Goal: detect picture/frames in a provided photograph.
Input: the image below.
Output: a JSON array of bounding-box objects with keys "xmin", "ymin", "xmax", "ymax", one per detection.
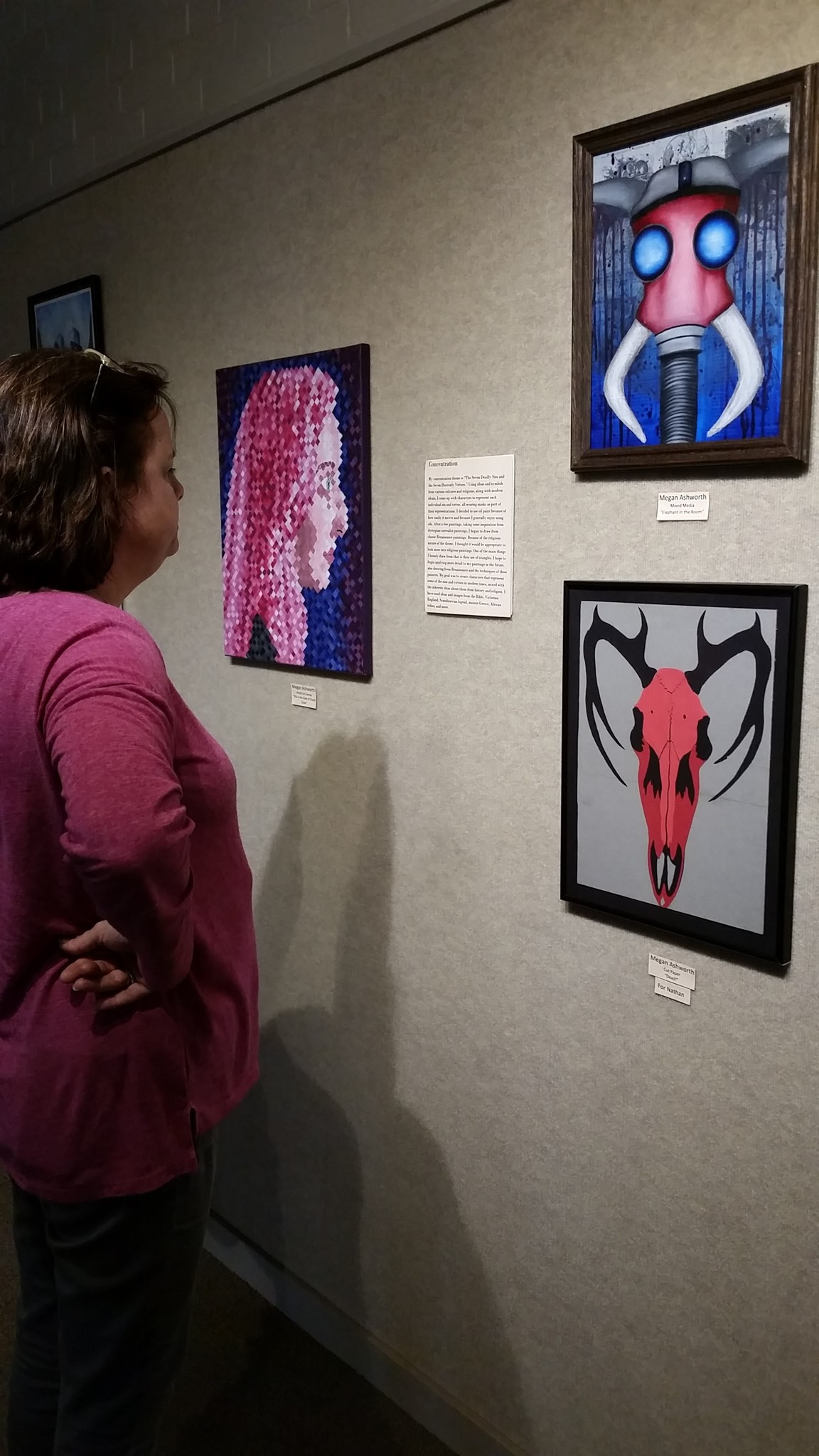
[
  {"xmin": 28, "ymin": 274, "xmax": 106, "ymax": 356},
  {"xmin": 558, "ymin": 578, "xmax": 809, "ymax": 967},
  {"xmin": 572, "ymin": 62, "xmax": 819, "ymax": 473}
]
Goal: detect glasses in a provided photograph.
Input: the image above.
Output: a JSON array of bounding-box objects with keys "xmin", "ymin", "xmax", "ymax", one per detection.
[{"xmin": 82, "ymin": 346, "xmax": 130, "ymax": 398}]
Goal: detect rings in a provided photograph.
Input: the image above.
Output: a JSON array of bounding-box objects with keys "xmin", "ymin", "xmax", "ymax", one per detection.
[{"xmin": 126, "ymin": 969, "xmax": 134, "ymax": 984}]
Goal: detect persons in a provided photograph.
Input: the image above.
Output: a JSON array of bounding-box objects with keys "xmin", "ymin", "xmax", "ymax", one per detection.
[{"xmin": 0, "ymin": 341, "xmax": 262, "ymax": 1456}]
